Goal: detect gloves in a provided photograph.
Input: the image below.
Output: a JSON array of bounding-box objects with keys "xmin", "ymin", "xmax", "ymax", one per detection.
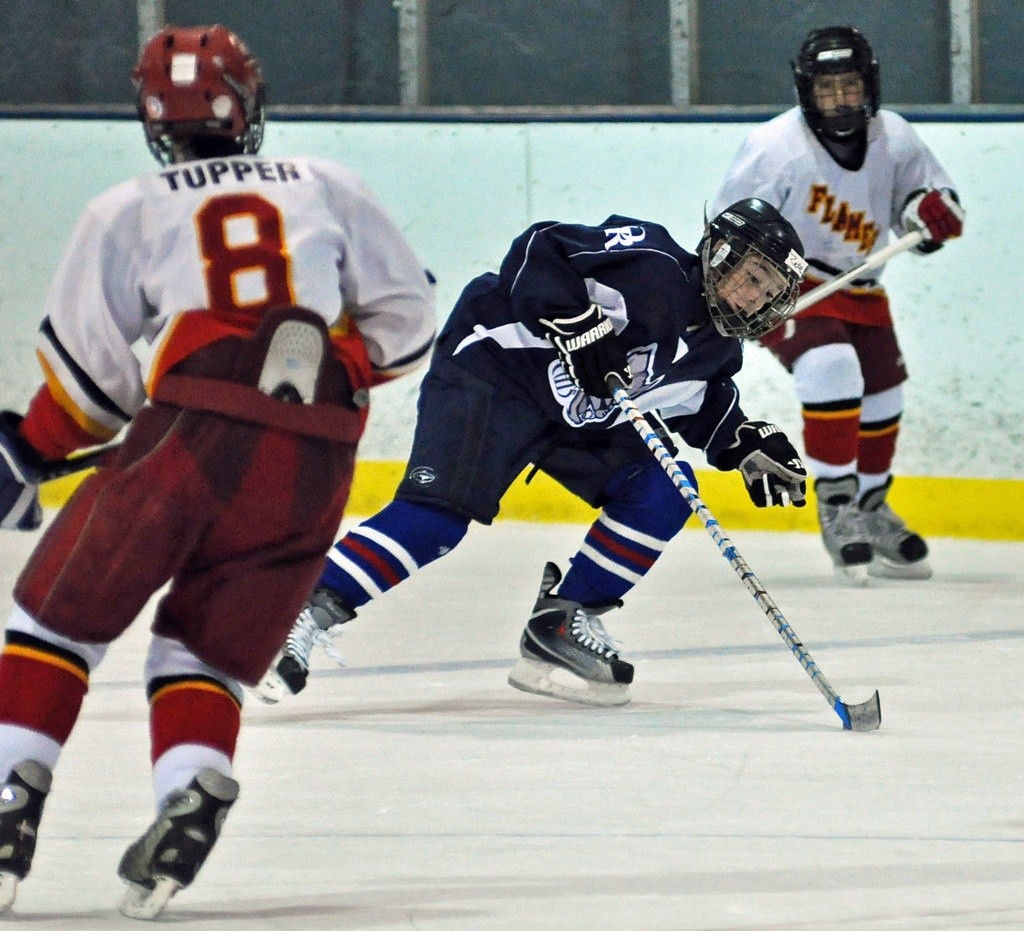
[
  {"xmin": 538, "ymin": 301, "xmax": 634, "ymax": 399},
  {"xmin": 915, "ymin": 188, "xmax": 964, "ymax": 241},
  {"xmin": 0, "ymin": 410, "xmax": 42, "ymax": 531},
  {"xmin": 724, "ymin": 421, "xmax": 807, "ymax": 508}
]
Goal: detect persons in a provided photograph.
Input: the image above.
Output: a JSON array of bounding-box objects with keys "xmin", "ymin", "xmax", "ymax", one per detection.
[
  {"xmin": 0, "ymin": 24, "xmax": 438, "ymax": 918},
  {"xmin": 706, "ymin": 24, "xmax": 965, "ymax": 586},
  {"xmin": 240, "ymin": 197, "xmax": 806, "ymax": 708}
]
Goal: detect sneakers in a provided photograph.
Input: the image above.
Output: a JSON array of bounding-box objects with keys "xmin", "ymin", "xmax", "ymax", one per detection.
[
  {"xmin": 508, "ymin": 562, "xmax": 633, "ymax": 706},
  {"xmin": 116, "ymin": 768, "xmax": 239, "ymax": 917},
  {"xmin": 815, "ymin": 474, "xmax": 875, "ymax": 587},
  {"xmin": 0, "ymin": 759, "xmax": 52, "ymax": 912},
  {"xmin": 240, "ymin": 579, "xmax": 357, "ymax": 705},
  {"xmin": 856, "ymin": 474, "xmax": 934, "ymax": 580}
]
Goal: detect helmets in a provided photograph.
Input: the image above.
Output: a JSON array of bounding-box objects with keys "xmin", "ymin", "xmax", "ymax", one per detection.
[
  {"xmin": 791, "ymin": 26, "xmax": 879, "ymax": 131},
  {"xmin": 695, "ymin": 197, "xmax": 808, "ymax": 335},
  {"xmin": 132, "ymin": 24, "xmax": 265, "ymax": 165}
]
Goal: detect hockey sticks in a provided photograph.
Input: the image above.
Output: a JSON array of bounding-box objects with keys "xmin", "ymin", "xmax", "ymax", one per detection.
[
  {"xmin": 786, "ymin": 229, "xmax": 921, "ymax": 317},
  {"xmin": 34, "ymin": 443, "xmax": 122, "ymax": 486},
  {"xmin": 607, "ymin": 369, "xmax": 884, "ymax": 733}
]
